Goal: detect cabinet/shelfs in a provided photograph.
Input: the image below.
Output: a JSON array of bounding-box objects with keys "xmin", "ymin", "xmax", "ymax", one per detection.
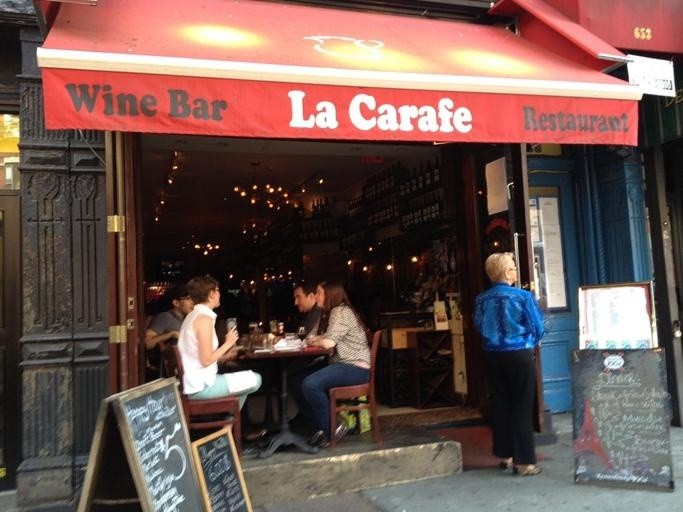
[
  {"xmin": 379, "ymin": 312, "xmax": 433, "ymax": 407},
  {"xmin": 407, "ymin": 330, "xmax": 457, "ymax": 409}
]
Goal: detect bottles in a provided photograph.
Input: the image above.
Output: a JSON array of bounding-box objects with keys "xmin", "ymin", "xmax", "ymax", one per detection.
[
  {"xmin": 297, "ymin": 156, "xmax": 443, "ymax": 249},
  {"xmin": 278, "ymin": 321, "xmax": 285, "ymax": 337}
]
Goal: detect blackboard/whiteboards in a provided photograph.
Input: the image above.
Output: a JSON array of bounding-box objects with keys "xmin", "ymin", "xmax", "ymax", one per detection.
[
  {"xmin": 570, "ymin": 347, "xmax": 674, "ymax": 492},
  {"xmin": 112, "ymin": 376, "xmax": 207, "ymax": 511},
  {"xmin": 191, "ymin": 424, "xmax": 254, "ymax": 512}
]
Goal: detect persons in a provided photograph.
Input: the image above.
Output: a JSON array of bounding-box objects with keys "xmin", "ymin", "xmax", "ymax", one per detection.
[
  {"xmin": 144, "ymin": 286, "xmax": 269, "ymax": 441},
  {"xmin": 299, "ymin": 281, "xmax": 371, "ymax": 449},
  {"xmin": 284, "ymin": 279, "xmax": 324, "ymax": 420},
  {"xmin": 473, "ymin": 251, "xmax": 546, "ymax": 475},
  {"xmin": 178, "ymin": 277, "xmax": 263, "ymax": 430}
]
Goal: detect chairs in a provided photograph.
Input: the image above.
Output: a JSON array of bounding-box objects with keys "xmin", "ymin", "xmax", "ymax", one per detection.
[
  {"xmin": 172, "ymin": 344, "xmax": 242, "ymax": 460},
  {"xmin": 329, "ymin": 330, "xmax": 382, "ymax": 447},
  {"xmin": 145, "ymin": 342, "xmax": 164, "ymax": 375}
]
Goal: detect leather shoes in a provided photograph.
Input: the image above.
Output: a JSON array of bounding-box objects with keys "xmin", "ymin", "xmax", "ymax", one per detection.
[
  {"xmin": 310, "ymin": 429, "xmax": 324, "ymax": 446},
  {"xmin": 513, "ymin": 464, "xmax": 541, "ymax": 476},
  {"xmin": 321, "ymin": 424, "xmax": 347, "ymax": 447},
  {"xmin": 501, "ymin": 458, "xmax": 513, "ymax": 471}
]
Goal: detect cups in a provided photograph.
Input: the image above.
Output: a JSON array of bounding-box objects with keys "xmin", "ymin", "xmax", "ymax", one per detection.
[
  {"xmin": 225, "ymin": 318, "xmax": 240, "ymax": 347},
  {"xmin": 424, "ymin": 319, "xmax": 434, "ymax": 331},
  {"xmin": 286, "ymin": 333, "xmax": 296, "ymax": 347},
  {"xmin": 248, "ymin": 321, "xmax": 259, "ymax": 342},
  {"xmin": 269, "ymin": 320, "xmax": 278, "ymax": 336},
  {"xmin": 262, "ymin": 336, "xmax": 272, "ymax": 351}
]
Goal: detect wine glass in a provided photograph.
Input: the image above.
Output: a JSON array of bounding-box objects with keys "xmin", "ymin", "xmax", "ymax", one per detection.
[{"xmin": 298, "ymin": 327, "xmax": 308, "ymax": 345}]
